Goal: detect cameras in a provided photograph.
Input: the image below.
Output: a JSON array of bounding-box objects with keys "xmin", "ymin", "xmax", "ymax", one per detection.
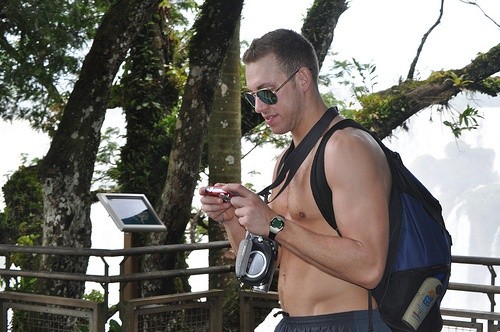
[{"xmin": 236, "ymin": 235, "xmax": 280, "ymax": 294}]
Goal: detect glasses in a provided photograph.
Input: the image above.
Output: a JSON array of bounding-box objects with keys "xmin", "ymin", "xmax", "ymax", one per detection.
[{"xmin": 244, "ymin": 69, "xmax": 301, "ymax": 108}]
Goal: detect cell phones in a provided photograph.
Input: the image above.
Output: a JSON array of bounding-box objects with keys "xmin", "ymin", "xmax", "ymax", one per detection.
[{"xmin": 205, "ymin": 187, "xmax": 241, "ymax": 202}]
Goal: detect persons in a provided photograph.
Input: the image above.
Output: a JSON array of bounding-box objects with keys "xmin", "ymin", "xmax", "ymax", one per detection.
[{"xmin": 199, "ymin": 29, "xmax": 392, "ymax": 332}]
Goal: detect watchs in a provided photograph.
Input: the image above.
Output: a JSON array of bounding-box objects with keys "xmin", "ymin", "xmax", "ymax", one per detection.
[{"xmin": 268, "ymin": 214, "xmax": 285, "ymax": 240}]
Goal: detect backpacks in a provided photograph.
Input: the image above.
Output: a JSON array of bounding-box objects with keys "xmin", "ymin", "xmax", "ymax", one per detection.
[{"xmin": 310, "ymin": 118, "xmax": 453, "ymax": 332}]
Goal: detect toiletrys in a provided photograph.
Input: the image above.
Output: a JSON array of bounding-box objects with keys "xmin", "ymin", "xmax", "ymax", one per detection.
[{"xmin": 402, "ymin": 274, "xmax": 445, "ymax": 330}]
